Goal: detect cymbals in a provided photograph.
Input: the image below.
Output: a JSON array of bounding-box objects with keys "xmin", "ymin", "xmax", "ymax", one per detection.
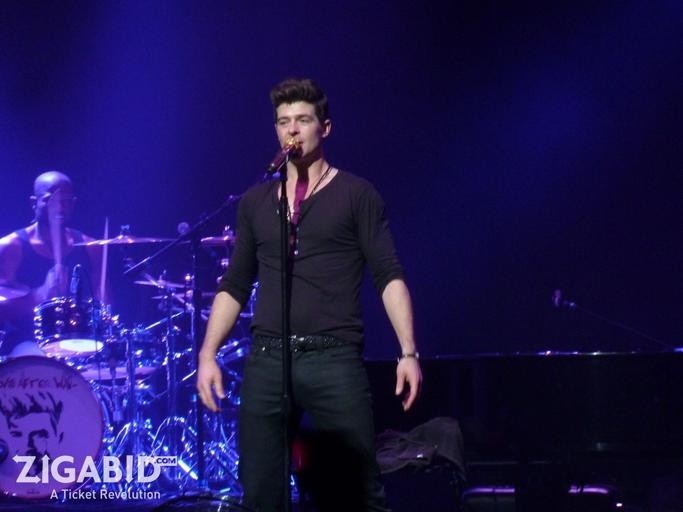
[
  {"xmin": 152, "ymin": 292, "xmax": 215, "ymax": 299},
  {"xmin": 72, "ymin": 236, "xmax": 174, "ymax": 246},
  {"xmin": 182, "ymin": 235, "xmax": 237, "ymax": 245},
  {"xmin": 134, "ymin": 279, "xmax": 186, "ymax": 288}
]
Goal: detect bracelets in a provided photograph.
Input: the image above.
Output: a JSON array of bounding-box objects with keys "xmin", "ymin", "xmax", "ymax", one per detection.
[{"xmin": 396, "ymin": 350, "xmax": 421, "ymax": 360}]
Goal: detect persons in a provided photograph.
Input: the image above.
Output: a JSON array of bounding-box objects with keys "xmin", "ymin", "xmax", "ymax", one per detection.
[
  {"xmin": 0, "ymin": 169, "xmax": 109, "ymax": 357},
  {"xmin": 193, "ymin": 78, "xmax": 424, "ymax": 511}
]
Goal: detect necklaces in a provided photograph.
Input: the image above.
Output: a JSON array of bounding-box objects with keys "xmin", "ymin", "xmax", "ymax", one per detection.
[{"xmin": 269, "ymin": 163, "xmax": 331, "ymax": 228}]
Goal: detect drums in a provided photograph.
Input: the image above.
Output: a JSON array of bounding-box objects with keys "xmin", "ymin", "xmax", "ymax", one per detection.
[
  {"xmin": 35, "ymin": 297, "xmax": 106, "ymax": 357},
  {"xmin": 1, "ymin": 353, "xmax": 105, "ymax": 501},
  {"xmin": 69, "ymin": 324, "xmax": 165, "ymax": 380}
]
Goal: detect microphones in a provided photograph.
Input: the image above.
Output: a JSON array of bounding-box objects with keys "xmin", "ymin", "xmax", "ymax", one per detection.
[
  {"xmin": 552, "ymin": 289, "xmax": 563, "ymax": 308},
  {"xmin": 259, "ymin": 138, "xmax": 301, "ymax": 185},
  {"xmin": 69, "ymin": 264, "xmax": 82, "ymax": 294}
]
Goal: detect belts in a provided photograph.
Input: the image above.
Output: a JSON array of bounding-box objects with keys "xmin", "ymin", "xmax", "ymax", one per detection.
[{"xmin": 257, "ymin": 335, "xmax": 338, "ymax": 352}]
